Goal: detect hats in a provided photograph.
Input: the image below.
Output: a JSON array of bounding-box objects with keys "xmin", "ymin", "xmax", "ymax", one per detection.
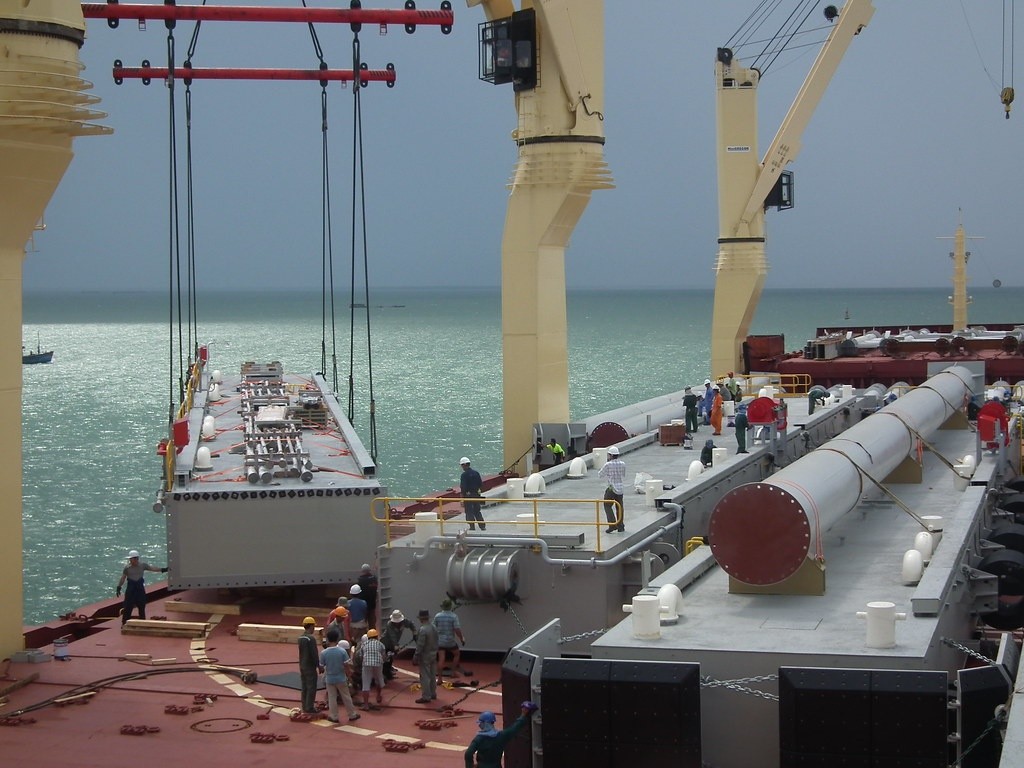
[
  {"xmin": 126, "ymin": 550, "xmax": 138, "ymax": 559},
  {"xmin": 336, "ymin": 597, "xmax": 350, "ymax": 607},
  {"xmin": 417, "ymin": 609, "xmax": 430, "ymax": 618},
  {"xmin": 739, "ymin": 405, "xmax": 746, "ymax": 410},
  {"xmin": 390, "ymin": 610, "xmax": 404, "ymax": 623},
  {"xmin": 706, "ymin": 440, "xmax": 714, "ymax": 447},
  {"xmin": 728, "ymin": 372, "xmax": 734, "ymax": 375}
]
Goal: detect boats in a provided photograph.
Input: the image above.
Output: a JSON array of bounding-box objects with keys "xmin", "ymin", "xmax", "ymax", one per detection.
[{"xmin": 21, "ymin": 346, "xmax": 55, "ymax": 364}]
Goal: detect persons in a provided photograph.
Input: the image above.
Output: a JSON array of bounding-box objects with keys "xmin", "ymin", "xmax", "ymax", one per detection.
[
  {"xmin": 297, "ymin": 617, "xmax": 324, "ymax": 713},
  {"xmin": 598, "ymin": 446, "xmax": 625, "ymax": 533},
  {"xmin": 116, "ymin": 550, "xmax": 172, "ymax": 629},
  {"xmin": 682, "ymin": 386, "xmax": 699, "ymax": 433},
  {"xmin": 320, "ymin": 564, "xmax": 418, "ymax": 711},
  {"xmin": 412, "ymin": 609, "xmax": 439, "ymax": 703},
  {"xmin": 734, "ymin": 405, "xmax": 752, "ymax": 454},
  {"xmin": 710, "ymin": 385, "xmax": 723, "ymax": 436},
  {"xmin": 432, "ymin": 599, "xmax": 465, "ymax": 685},
  {"xmin": 716, "ymin": 372, "xmax": 743, "ymax": 404},
  {"xmin": 464, "ymin": 705, "xmax": 531, "ymax": 768},
  {"xmin": 808, "ymin": 389, "xmax": 830, "ymax": 415},
  {"xmin": 698, "ymin": 380, "xmax": 716, "ymax": 425},
  {"xmin": 538, "ymin": 438, "xmax": 565, "ymax": 466},
  {"xmin": 319, "ymin": 630, "xmax": 361, "ymax": 722},
  {"xmin": 700, "ymin": 440, "xmax": 718, "ymax": 468},
  {"xmin": 459, "ymin": 457, "xmax": 487, "ymax": 531}
]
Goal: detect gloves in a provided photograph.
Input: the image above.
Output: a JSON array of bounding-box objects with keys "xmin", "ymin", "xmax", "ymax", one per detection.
[
  {"xmin": 116, "ymin": 586, "xmax": 121, "ymax": 597},
  {"xmin": 161, "ymin": 567, "xmax": 168, "ymax": 572}
]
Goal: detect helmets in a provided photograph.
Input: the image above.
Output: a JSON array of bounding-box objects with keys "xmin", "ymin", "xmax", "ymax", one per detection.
[
  {"xmin": 337, "ymin": 640, "xmax": 350, "ymax": 649},
  {"xmin": 608, "ymin": 447, "xmax": 620, "ymax": 455},
  {"xmin": 460, "ymin": 457, "xmax": 470, "ymax": 464},
  {"xmin": 367, "ymin": 629, "xmax": 379, "ymax": 638},
  {"xmin": 335, "ymin": 606, "xmax": 348, "ymax": 618},
  {"xmin": 303, "ymin": 617, "xmax": 318, "ymax": 626},
  {"xmin": 713, "ymin": 386, "xmax": 719, "ymax": 390},
  {"xmin": 705, "ymin": 380, "xmax": 710, "ymax": 384},
  {"xmin": 361, "ymin": 634, "xmax": 368, "ymax": 642},
  {"xmin": 350, "ymin": 585, "xmax": 363, "ymax": 594}
]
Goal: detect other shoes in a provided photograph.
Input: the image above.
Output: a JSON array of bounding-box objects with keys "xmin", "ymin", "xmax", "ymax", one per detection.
[
  {"xmin": 308, "ymin": 667, "xmax": 438, "ymax": 722},
  {"xmin": 606, "ymin": 526, "xmax": 618, "ymax": 533},
  {"xmin": 618, "ymin": 527, "xmax": 625, "ymax": 532}
]
